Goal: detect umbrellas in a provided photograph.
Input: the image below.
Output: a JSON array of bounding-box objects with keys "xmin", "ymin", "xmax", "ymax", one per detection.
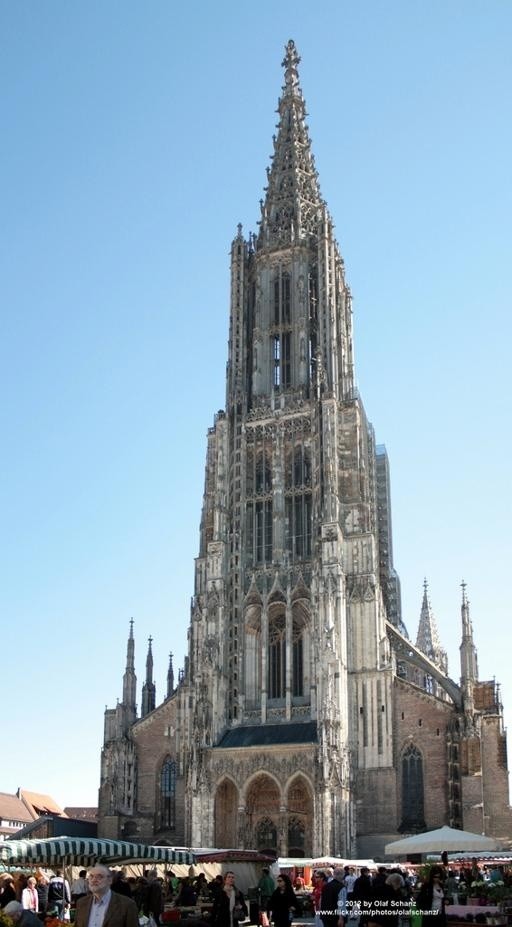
[{"xmin": 384, "ymin": 825, "xmax": 498, "ymax": 864}]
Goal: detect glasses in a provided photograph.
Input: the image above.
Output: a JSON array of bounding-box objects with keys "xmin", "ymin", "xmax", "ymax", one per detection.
[
  {"xmin": 276, "ymin": 879, "xmax": 286, "ymax": 884},
  {"xmin": 88, "ymin": 874, "xmax": 109, "ymax": 881}
]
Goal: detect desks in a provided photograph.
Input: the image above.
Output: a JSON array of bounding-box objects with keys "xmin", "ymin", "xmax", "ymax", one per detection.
[{"xmin": 445, "ymin": 905, "xmax": 500, "ymax": 918}]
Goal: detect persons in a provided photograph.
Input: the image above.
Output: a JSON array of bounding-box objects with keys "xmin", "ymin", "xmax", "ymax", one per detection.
[{"xmin": 1, "ymin": 862, "xmax": 512, "ymax": 927}]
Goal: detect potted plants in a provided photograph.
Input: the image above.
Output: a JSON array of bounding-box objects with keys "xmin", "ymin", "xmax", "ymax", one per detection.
[{"xmin": 443, "ymin": 878, "xmax": 512, "ymax": 910}]
